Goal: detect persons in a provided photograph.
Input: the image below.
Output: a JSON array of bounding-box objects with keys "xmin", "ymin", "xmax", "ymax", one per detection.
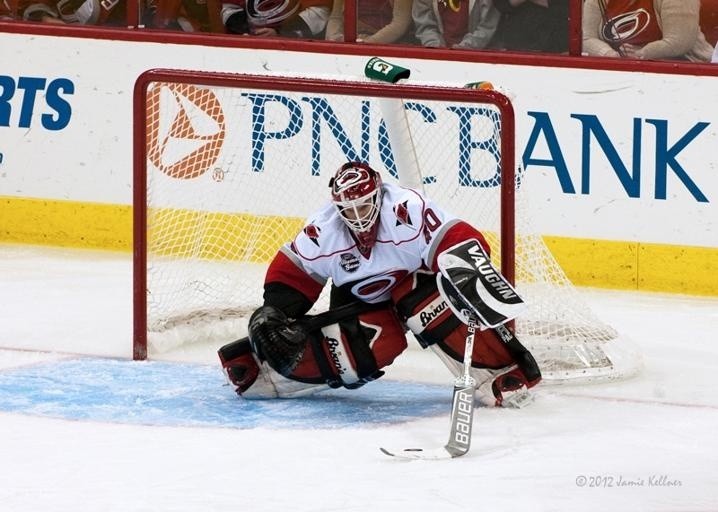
[
  {"xmin": 216, "ymin": 162, "xmax": 543, "ymax": 412},
  {"xmin": 0, "ymin": 0, "xmax": 718, "ymax": 68}
]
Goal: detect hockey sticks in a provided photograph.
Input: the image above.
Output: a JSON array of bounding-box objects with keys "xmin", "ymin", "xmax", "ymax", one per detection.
[{"xmin": 378, "ymin": 313, "xmax": 477, "ymax": 461}]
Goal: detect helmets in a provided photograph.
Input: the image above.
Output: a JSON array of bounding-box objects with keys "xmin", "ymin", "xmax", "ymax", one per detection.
[{"xmin": 331, "ymin": 163, "xmax": 381, "ymax": 248}]
